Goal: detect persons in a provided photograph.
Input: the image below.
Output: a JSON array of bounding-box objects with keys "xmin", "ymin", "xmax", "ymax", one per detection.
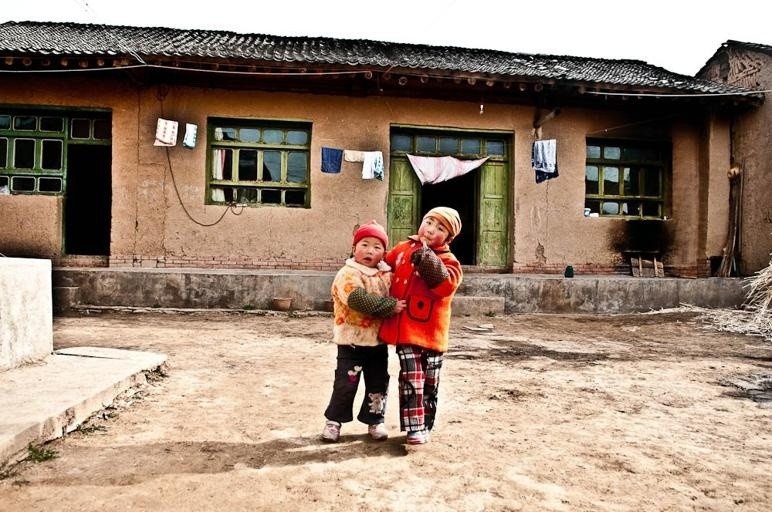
[
  {"xmin": 384, "ymin": 206, "xmax": 463, "ymax": 444},
  {"xmin": 322, "ymin": 221, "xmax": 407, "ymax": 441}
]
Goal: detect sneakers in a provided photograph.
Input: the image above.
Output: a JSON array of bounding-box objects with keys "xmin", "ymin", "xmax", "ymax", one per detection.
[
  {"xmin": 406, "ymin": 430, "xmax": 431, "ymax": 444},
  {"xmin": 367, "ymin": 423, "xmax": 388, "ymax": 440},
  {"xmin": 322, "ymin": 421, "xmax": 341, "ymax": 442}
]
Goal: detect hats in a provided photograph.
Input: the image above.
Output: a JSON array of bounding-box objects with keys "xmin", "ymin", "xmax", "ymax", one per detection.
[
  {"xmin": 423, "ymin": 207, "xmax": 462, "ymax": 236},
  {"xmin": 353, "ymin": 220, "xmax": 388, "ymax": 250}
]
